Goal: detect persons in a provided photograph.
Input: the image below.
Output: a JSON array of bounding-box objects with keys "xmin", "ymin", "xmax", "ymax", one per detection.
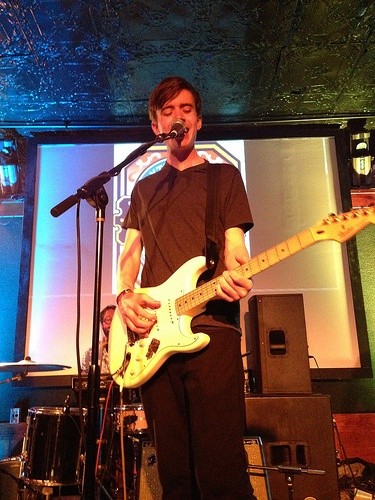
[
  {"xmin": 116, "ymin": 77, "xmax": 258, "ymax": 500},
  {"xmin": 62, "ymin": 305, "xmax": 118, "ymax": 478}
]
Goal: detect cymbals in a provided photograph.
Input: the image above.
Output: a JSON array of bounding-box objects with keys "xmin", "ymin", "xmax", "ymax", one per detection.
[{"xmin": 0, "ymin": 356, "xmax": 72, "ymax": 373}]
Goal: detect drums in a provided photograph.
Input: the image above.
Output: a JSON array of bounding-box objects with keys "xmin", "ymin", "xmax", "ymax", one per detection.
[
  {"xmin": 111, "ymin": 386, "xmax": 150, "ymax": 433},
  {"xmin": 19, "ymin": 407, "xmax": 88, "ymax": 486}
]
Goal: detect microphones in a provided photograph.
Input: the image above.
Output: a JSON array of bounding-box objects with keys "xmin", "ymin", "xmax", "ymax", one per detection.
[{"xmin": 169, "ymin": 119, "xmax": 184, "ymax": 138}]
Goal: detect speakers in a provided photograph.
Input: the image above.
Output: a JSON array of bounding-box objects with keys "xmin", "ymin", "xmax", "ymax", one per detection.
[
  {"xmin": 115, "ymin": 429, "xmax": 270, "ymax": 500},
  {"xmin": 248, "ymin": 292, "xmax": 313, "ymax": 396},
  {"xmin": 242, "ymin": 393, "xmax": 340, "ymax": 500}
]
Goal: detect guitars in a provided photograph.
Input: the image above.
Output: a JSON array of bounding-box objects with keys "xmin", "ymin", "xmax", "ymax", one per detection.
[{"xmin": 108, "ymin": 208, "xmax": 375, "ymax": 385}]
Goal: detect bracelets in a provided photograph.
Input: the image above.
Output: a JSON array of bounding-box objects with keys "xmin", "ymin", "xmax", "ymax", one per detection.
[{"xmin": 116, "ymin": 288, "xmax": 133, "ymax": 305}]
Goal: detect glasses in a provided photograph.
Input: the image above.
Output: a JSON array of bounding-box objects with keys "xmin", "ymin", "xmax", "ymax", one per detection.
[{"xmin": 101, "ymin": 319, "xmax": 111, "ymax": 324}]
